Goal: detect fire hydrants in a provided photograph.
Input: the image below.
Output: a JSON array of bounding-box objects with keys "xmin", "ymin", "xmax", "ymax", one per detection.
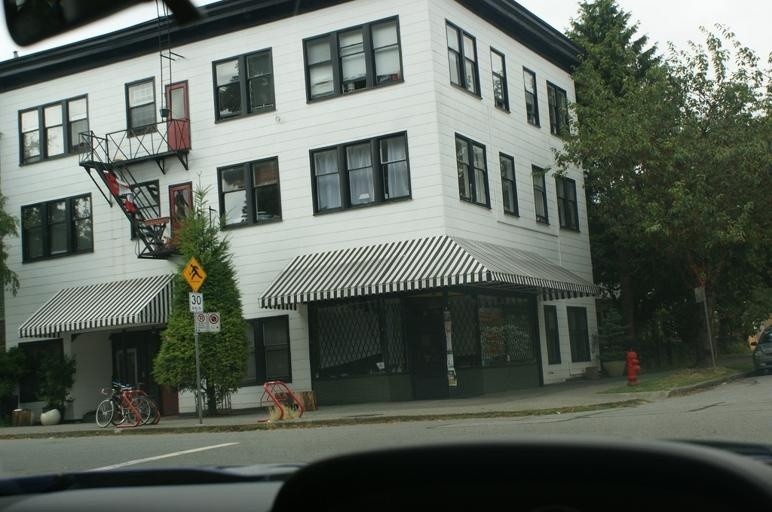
[{"xmin": 627, "ymin": 351, "xmax": 641, "ymax": 386}]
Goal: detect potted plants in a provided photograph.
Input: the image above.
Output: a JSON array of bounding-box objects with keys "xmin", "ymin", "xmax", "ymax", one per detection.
[{"xmin": 0, "ymin": 343, "xmax": 79, "ymax": 427}]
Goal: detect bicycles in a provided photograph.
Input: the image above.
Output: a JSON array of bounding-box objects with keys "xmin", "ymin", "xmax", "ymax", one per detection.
[{"xmin": 95, "ymin": 380, "xmax": 161, "ymax": 428}]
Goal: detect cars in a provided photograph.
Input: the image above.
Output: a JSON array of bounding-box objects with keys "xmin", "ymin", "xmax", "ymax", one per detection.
[{"xmin": 752, "ymin": 324, "xmax": 772, "ymax": 375}]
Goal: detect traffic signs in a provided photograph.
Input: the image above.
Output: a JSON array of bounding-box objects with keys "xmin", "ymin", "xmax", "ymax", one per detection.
[{"xmin": 188, "ymin": 292, "xmax": 204, "ymax": 314}]
